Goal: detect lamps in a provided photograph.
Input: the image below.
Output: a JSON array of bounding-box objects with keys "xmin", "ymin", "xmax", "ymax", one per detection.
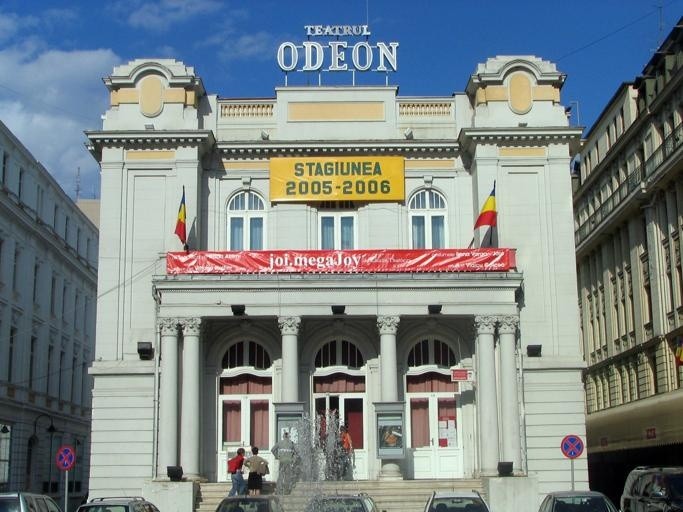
[
  {"xmin": 231, "ymin": 303, "xmax": 247, "ymax": 316},
  {"xmin": 496, "ymin": 462, "xmax": 515, "ymax": 477},
  {"xmin": 527, "ymin": 344, "xmax": 542, "ymax": 354},
  {"xmin": 426, "ymin": 303, "xmax": 443, "ymax": 314},
  {"xmin": 331, "ymin": 305, "xmax": 347, "ymax": 315},
  {"xmin": 136, "ymin": 341, "xmax": 153, "ymax": 360},
  {"xmin": 165, "ymin": 465, "xmax": 185, "ymax": 482},
  {"xmin": 30, "ymin": 411, "xmax": 58, "ymax": 444}
]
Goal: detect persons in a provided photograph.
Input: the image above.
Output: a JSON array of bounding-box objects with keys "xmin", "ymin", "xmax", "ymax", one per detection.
[
  {"xmin": 243, "ymin": 447, "xmax": 267, "ymax": 496},
  {"xmin": 271, "ymin": 433, "xmax": 299, "ymax": 495},
  {"xmin": 338, "ymin": 426, "xmax": 352, "ymax": 456},
  {"xmin": 227, "ymin": 448, "xmax": 247, "ymax": 496}
]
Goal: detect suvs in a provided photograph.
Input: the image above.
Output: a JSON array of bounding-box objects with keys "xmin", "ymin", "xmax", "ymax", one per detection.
[{"xmin": 619, "ymin": 465, "xmax": 682, "ymax": 512}]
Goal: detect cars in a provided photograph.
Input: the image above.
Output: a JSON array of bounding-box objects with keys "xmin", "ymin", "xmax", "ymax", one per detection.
[
  {"xmin": 538, "ymin": 490, "xmax": 621, "ymax": 512},
  {"xmin": 302, "ymin": 492, "xmax": 386, "ymax": 511},
  {"xmin": 0, "ymin": 492, "xmax": 62, "ymax": 511},
  {"xmin": 214, "ymin": 495, "xmax": 284, "ymax": 511},
  {"xmin": 75, "ymin": 495, "xmax": 159, "ymax": 512},
  {"xmin": 423, "ymin": 489, "xmax": 489, "ymax": 511}
]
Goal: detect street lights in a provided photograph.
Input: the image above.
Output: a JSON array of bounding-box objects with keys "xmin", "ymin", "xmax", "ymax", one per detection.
[
  {"xmin": 71, "ymin": 439, "xmax": 80, "ymax": 496},
  {"xmin": 28, "ymin": 414, "xmax": 55, "ymax": 494}
]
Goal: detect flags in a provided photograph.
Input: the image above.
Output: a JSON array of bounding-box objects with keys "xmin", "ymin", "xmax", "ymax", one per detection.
[
  {"xmin": 173, "ymin": 184, "xmax": 187, "ymax": 245},
  {"xmin": 472, "ymin": 180, "xmax": 496, "ymax": 249}
]
